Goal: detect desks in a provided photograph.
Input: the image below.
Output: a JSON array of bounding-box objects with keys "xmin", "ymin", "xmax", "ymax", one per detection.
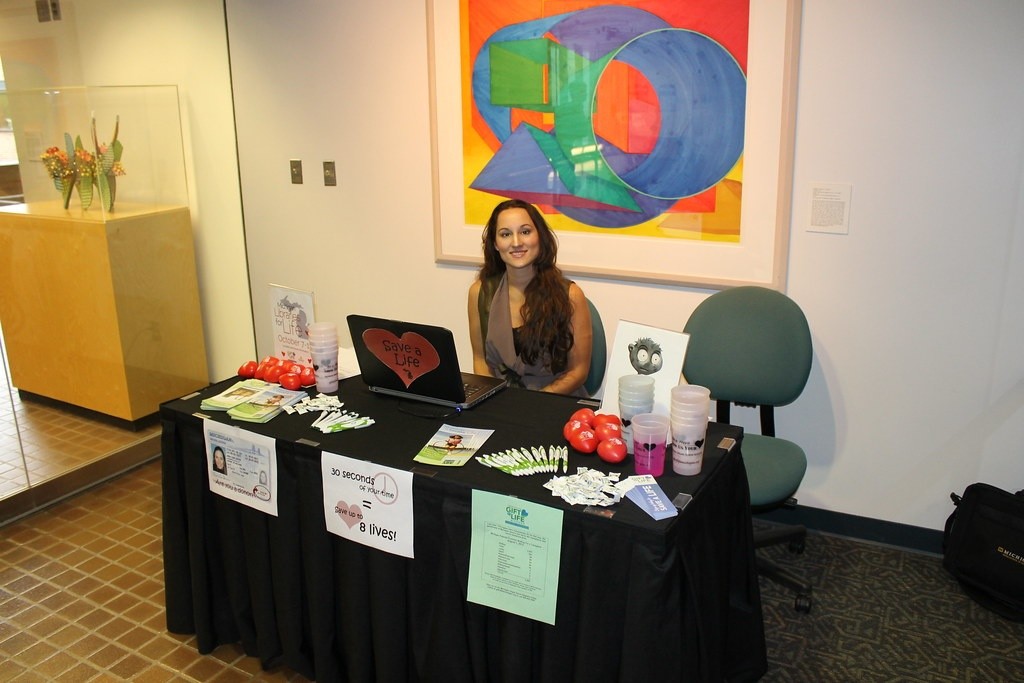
[{"xmin": 158, "ymin": 369, "xmax": 769, "ymax": 682}]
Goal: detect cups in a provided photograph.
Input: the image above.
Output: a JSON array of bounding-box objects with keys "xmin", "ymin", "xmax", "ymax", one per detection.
[
  {"xmin": 669, "ymin": 383, "xmax": 711, "ymax": 475},
  {"xmin": 617, "ymin": 375, "xmax": 656, "ymax": 455},
  {"xmin": 631, "ymin": 412, "xmax": 670, "ymax": 479},
  {"xmin": 308, "ymin": 322, "xmax": 339, "ymax": 392}
]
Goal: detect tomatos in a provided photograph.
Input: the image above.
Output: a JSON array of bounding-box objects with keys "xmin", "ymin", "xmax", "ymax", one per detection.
[
  {"xmin": 564, "ymin": 408, "xmax": 627, "ymax": 463},
  {"xmin": 237, "ymin": 355, "xmax": 315, "ymax": 390}
]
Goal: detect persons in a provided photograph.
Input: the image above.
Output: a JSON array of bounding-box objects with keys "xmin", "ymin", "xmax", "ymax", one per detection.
[
  {"xmin": 467, "ymin": 199, "xmax": 594, "ymax": 396},
  {"xmin": 211, "ymin": 446, "xmax": 226, "ymax": 475}
]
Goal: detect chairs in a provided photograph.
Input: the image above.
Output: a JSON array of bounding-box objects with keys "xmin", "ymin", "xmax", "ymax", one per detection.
[{"xmin": 681, "ymin": 285, "xmax": 812, "ymax": 612}]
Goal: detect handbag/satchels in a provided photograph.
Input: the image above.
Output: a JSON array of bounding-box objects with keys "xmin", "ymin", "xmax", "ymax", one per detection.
[{"xmin": 942, "ymin": 482, "xmax": 1024, "ymax": 623}]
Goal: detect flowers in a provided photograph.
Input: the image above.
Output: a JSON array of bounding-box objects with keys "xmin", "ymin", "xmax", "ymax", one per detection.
[{"xmin": 42, "ymin": 145, "xmax": 126, "ymax": 179}]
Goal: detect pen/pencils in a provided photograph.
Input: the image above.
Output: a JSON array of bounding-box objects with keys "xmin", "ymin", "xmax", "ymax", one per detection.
[
  {"xmin": 310, "ymin": 405, "xmax": 376, "ymax": 434},
  {"xmin": 474, "ymin": 444, "xmax": 569, "ymax": 477}
]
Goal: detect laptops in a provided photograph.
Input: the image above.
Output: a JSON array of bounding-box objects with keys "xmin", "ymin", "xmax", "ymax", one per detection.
[{"xmin": 347, "ymin": 314, "xmax": 507, "ymax": 409}]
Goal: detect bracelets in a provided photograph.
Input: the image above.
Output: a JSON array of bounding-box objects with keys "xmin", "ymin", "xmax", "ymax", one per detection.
[{"xmin": 543, "ymin": 383, "xmax": 554, "ymax": 393}]
